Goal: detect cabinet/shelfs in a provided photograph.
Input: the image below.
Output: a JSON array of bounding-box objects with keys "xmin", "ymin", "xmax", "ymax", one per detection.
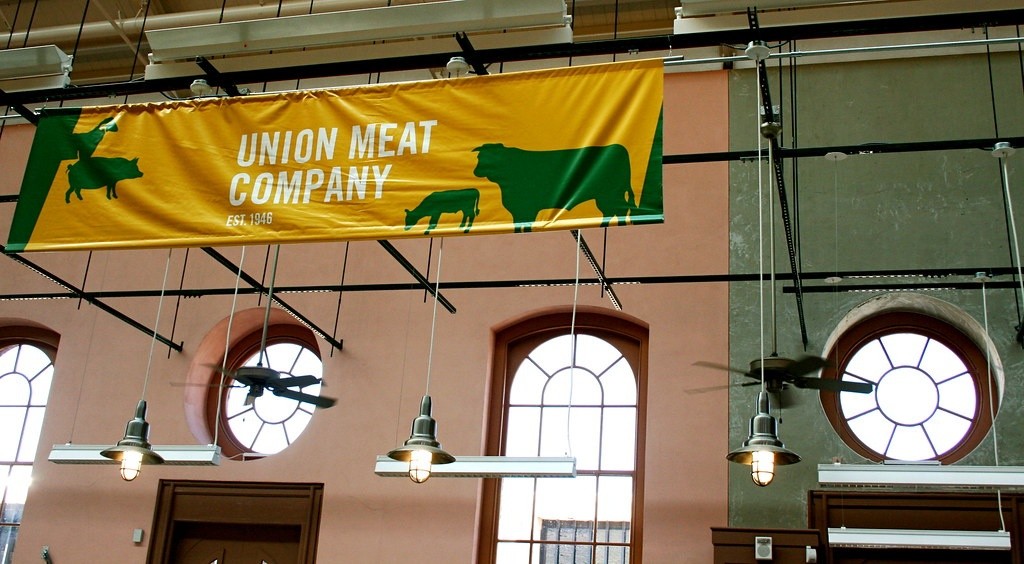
[
  {"xmin": 807, "ymin": 488, "xmax": 1024, "ymax": 564},
  {"xmin": 709, "ymin": 524, "xmax": 821, "ymax": 564}
]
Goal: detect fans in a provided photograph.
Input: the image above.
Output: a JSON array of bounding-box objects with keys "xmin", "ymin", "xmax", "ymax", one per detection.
[
  {"xmin": 167, "ymin": 361, "xmax": 338, "ymax": 424},
  {"xmin": 685, "ymin": 351, "xmax": 873, "ymax": 411}
]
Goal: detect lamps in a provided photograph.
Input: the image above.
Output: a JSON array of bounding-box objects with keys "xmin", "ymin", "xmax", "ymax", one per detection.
[
  {"xmin": 375, "ymin": 228, "xmax": 583, "ymax": 483},
  {"xmin": 817, "ymin": 463, "xmax": 1024, "ymax": 551},
  {"xmin": 723, "ymin": 40, "xmax": 803, "ymax": 487},
  {"xmin": 48, "ymin": 241, "xmax": 248, "ymax": 483}
]
